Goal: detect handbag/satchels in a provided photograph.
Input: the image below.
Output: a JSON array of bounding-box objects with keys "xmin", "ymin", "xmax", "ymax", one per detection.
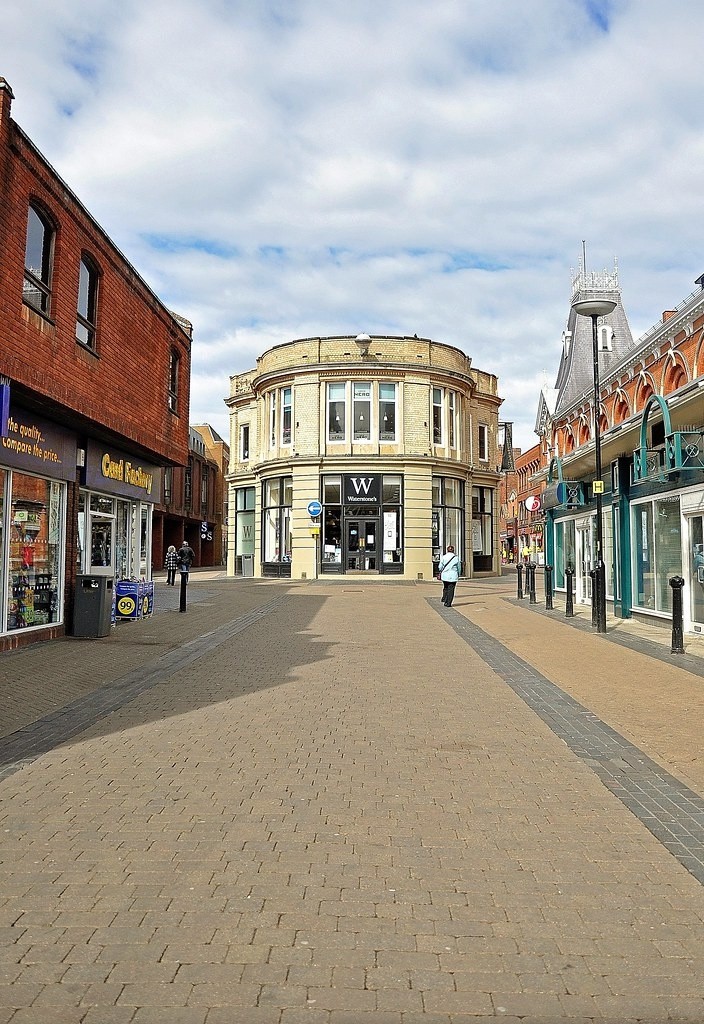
[
  {"xmin": 181, "ymin": 556, "xmax": 190, "ymax": 565},
  {"xmin": 437, "ymin": 573, "xmax": 441, "ymax": 580}
]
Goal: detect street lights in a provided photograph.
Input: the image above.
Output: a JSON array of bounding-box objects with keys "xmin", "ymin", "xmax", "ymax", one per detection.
[{"xmin": 573, "ymin": 298, "xmax": 618, "ymax": 633}]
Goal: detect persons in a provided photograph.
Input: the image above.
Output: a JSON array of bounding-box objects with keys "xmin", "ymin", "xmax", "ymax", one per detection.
[
  {"xmin": 178, "ymin": 540, "xmax": 195, "ymax": 584},
  {"xmin": 502, "ymin": 548, "xmax": 506, "ymax": 566},
  {"xmin": 163, "ymin": 545, "xmax": 181, "ymax": 587},
  {"xmin": 438, "ymin": 546, "xmax": 462, "ymax": 607},
  {"xmin": 523, "ymin": 545, "xmax": 531, "ymax": 563},
  {"xmin": 509, "ymin": 549, "xmax": 514, "ymax": 563}
]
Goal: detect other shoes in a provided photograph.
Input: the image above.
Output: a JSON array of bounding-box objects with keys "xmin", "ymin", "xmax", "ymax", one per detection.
[
  {"xmin": 444, "ymin": 603, "xmax": 451, "ymax": 607},
  {"xmin": 441, "ymin": 598, "xmax": 445, "ymax": 602}
]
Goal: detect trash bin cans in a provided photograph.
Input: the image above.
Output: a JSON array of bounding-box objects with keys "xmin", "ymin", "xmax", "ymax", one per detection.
[
  {"xmin": 63, "ymin": 573, "xmax": 115, "ymax": 639},
  {"xmin": 241, "ymin": 553, "xmax": 254, "ymax": 577}
]
